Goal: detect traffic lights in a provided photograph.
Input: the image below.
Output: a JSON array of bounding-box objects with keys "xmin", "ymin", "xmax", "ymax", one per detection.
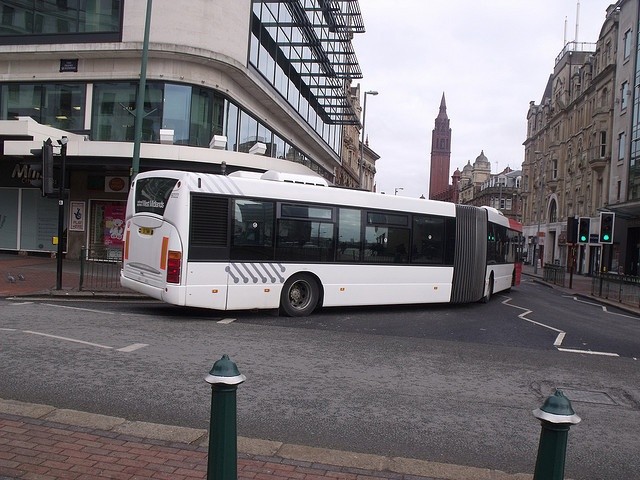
[
  {"xmin": 598, "ymin": 212, "xmax": 615, "ymax": 244},
  {"xmin": 578, "ymin": 217, "xmax": 591, "ymax": 244},
  {"xmin": 28, "ymin": 138, "xmax": 55, "ymax": 196},
  {"xmin": 567, "ymin": 217, "xmax": 578, "ymax": 243}
]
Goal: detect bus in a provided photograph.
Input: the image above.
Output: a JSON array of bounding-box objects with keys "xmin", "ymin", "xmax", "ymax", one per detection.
[{"xmin": 120, "ymin": 170, "xmax": 525, "ymax": 317}]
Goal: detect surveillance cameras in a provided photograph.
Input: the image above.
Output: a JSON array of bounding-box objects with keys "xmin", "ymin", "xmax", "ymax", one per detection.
[{"xmin": 57, "ymin": 138, "xmax": 68, "ymax": 145}]
